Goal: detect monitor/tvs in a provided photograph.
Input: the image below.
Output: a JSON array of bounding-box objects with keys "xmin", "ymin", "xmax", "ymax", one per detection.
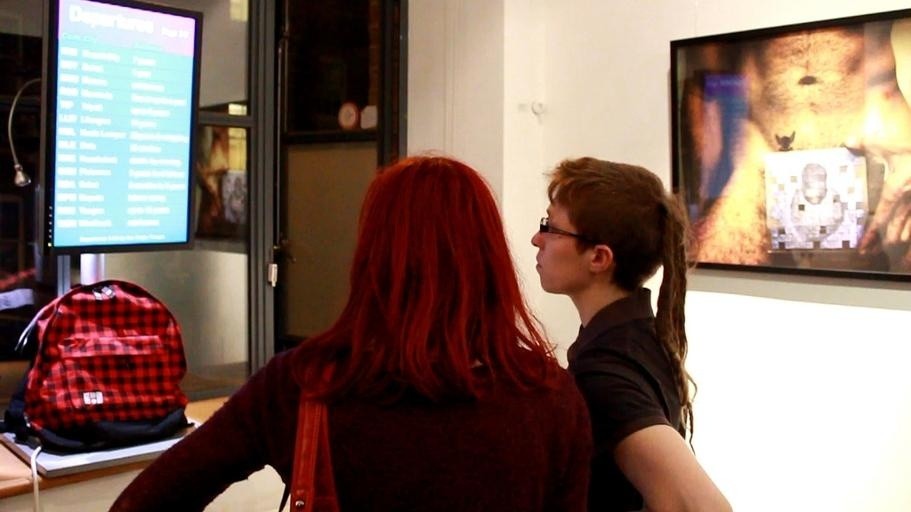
[{"xmin": 40, "ymin": 1, "xmax": 205, "ymax": 257}]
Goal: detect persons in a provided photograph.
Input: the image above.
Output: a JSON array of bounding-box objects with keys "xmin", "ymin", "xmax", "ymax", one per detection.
[
  {"xmin": 527, "ymin": 154, "xmax": 737, "ymax": 511},
  {"xmin": 104, "ymin": 151, "xmax": 600, "ymax": 511}
]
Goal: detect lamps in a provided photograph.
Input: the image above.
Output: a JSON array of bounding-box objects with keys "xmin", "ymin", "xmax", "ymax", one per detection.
[{"xmin": 8, "ymin": 77, "xmax": 42, "ymax": 188}]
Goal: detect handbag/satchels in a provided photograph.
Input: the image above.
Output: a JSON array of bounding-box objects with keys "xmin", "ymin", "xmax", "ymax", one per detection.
[{"xmin": 4, "ymin": 279, "xmax": 189, "ymax": 456}]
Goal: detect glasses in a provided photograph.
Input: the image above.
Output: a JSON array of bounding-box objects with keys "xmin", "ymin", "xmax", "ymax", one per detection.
[{"xmin": 539, "ymin": 215, "xmax": 602, "ymax": 245}]
